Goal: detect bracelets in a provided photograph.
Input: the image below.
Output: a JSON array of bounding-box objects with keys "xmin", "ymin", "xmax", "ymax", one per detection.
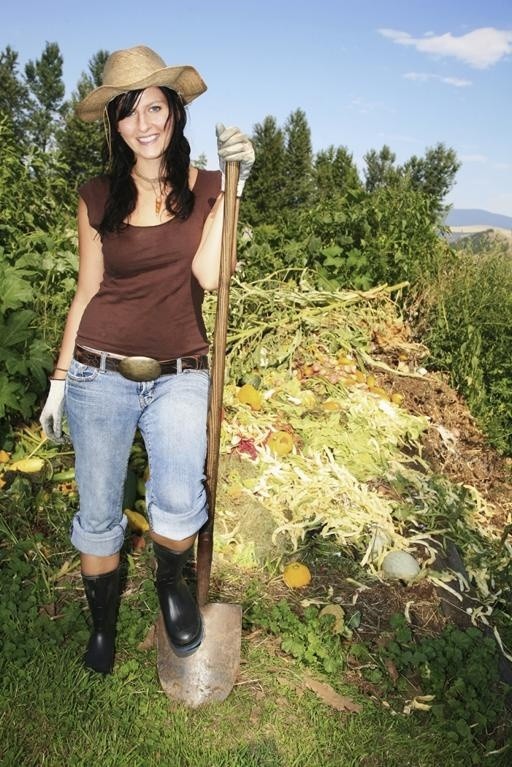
[{"xmin": 52, "ymin": 367, "xmax": 68, "ymax": 374}]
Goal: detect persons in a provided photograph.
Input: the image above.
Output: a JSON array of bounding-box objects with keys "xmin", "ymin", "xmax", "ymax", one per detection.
[{"xmin": 39, "ymin": 44, "xmax": 257, "ymax": 679}]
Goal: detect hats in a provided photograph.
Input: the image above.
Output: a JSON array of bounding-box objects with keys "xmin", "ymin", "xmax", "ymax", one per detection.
[{"xmin": 78, "ymin": 45, "xmax": 208, "ymax": 122}]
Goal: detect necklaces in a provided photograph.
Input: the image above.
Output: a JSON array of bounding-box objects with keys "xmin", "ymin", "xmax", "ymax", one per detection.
[{"xmin": 131, "ymin": 165, "xmax": 168, "ymax": 218}]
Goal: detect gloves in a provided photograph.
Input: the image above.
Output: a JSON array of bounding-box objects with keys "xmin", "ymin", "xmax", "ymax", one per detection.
[
  {"xmin": 215, "ymin": 122, "xmax": 255, "ymax": 198},
  {"xmin": 39, "ymin": 378, "xmax": 71, "ymax": 444}
]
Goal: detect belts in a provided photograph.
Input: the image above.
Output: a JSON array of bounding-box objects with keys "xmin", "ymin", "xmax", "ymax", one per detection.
[{"xmin": 73, "ymin": 343, "xmax": 208, "ymax": 375}]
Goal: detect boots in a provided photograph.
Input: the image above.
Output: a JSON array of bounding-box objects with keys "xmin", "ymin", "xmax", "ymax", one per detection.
[
  {"xmin": 81, "ymin": 565, "xmax": 121, "ymax": 677},
  {"xmin": 153, "ymin": 541, "xmax": 206, "ymax": 655}
]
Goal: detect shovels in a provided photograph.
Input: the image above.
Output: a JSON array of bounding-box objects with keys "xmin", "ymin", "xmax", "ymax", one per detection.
[{"xmin": 156, "ymin": 160, "xmax": 244, "ymax": 710}]
